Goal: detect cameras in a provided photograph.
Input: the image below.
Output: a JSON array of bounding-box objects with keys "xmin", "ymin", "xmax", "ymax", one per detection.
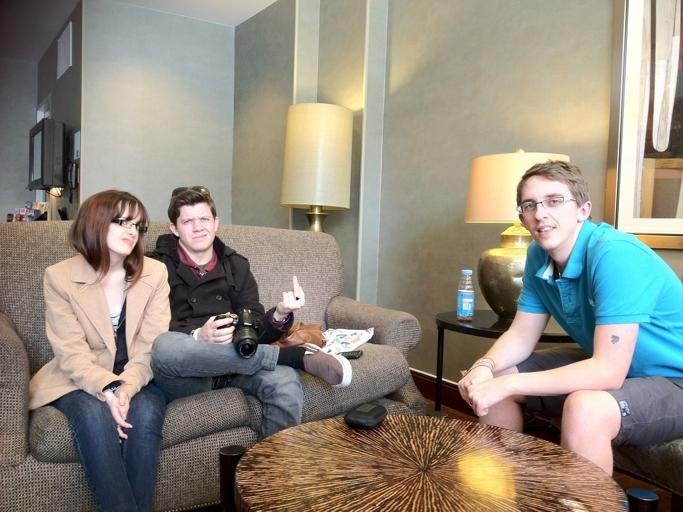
[{"xmin": 215, "ymin": 306, "xmax": 259, "ymax": 358}]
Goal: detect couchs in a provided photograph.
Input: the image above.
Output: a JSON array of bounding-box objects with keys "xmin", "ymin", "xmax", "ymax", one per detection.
[{"xmin": 0, "ymin": 219, "xmax": 422, "ymax": 512}]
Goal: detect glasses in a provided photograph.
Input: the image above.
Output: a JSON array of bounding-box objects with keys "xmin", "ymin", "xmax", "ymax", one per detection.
[
  {"xmin": 515, "ymin": 197, "xmax": 575, "ymax": 213},
  {"xmin": 171, "ymin": 185, "xmax": 210, "ymax": 199},
  {"xmin": 110, "ymin": 216, "xmax": 147, "ymax": 233}
]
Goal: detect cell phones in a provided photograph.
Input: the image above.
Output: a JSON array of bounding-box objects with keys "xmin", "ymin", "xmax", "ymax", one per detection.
[{"xmin": 338, "ymin": 350, "xmax": 363, "ymax": 359}]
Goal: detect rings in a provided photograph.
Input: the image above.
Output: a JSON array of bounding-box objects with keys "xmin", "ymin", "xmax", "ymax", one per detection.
[
  {"xmin": 296, "ymin": 297, "xmax": 301, "ymax": 300},
  {"xmin": 468, "ymin": 400, "xmax": 472, "ymax": 405}
]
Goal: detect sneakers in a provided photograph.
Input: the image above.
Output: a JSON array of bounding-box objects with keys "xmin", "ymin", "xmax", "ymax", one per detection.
[{"xmin": 299, "ymin": 342, "xmax": 353, "ymax": 389}]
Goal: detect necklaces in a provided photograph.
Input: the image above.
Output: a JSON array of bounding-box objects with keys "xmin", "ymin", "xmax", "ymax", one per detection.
[{"xmin": 555, "ymin": 262, "xmax": 565, "ymax": 279}]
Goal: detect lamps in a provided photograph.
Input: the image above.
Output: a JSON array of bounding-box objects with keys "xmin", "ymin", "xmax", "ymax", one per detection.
[
  {"xmin": 462, "ymin": 149, "xmax": 570, "ymax": 324},
  {"xmin": 279, "ymin": 102, "xmax": 352, "ymax": 233}
]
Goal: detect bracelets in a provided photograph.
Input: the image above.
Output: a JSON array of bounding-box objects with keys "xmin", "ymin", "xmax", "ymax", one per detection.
[{"xmin": 468, "ymin": 358, "xmax": 496, "ymax": 372}]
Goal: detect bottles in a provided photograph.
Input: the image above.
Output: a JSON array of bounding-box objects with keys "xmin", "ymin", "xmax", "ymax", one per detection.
[
  {"xmin": 7, "ymin": 214, "xmax": 13, "ymax": 222},
  {"xmin": 456, "ymin": 268, "xmax": 475, "ymax": 324},
  {"xmin": 15, "ymin": 214, "xmax": 23, "ymax": 222},
  {"xmin": 26, "ymin": 215, "xmax": 34, "ymax": 222}
]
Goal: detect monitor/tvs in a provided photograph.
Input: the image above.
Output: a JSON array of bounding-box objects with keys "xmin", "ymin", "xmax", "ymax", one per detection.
[{"xmin": 27, "ymin": 117, "xmax": 65, "ymax": 190}]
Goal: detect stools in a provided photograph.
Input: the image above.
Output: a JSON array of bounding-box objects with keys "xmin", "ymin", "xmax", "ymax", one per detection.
[{"xmin": 521, "ymin": 400, "xmax": 683, "ymax": 501}]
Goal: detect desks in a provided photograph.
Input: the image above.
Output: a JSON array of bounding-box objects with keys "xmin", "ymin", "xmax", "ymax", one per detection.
[{"xmin": 434, "ymin": 309, "xmax": 576, "ymax": 411}]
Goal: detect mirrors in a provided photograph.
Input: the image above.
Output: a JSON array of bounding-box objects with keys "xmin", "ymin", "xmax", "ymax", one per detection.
[{"xmin": 604, "ymin": 0, "xmax": 682, "ymax": 249}]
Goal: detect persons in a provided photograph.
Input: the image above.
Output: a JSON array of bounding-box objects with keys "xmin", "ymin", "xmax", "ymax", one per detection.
[
  {"xmin": 25, "ymin": 190, "xmax": 173, "ymax": 512},
  {"xmin": 457, "ymin": 160, "xmax": 683, "ymax": 478},
  {"xmin": 141, "ymin": 184, "xmax": 353, "ymax": 440}
]
